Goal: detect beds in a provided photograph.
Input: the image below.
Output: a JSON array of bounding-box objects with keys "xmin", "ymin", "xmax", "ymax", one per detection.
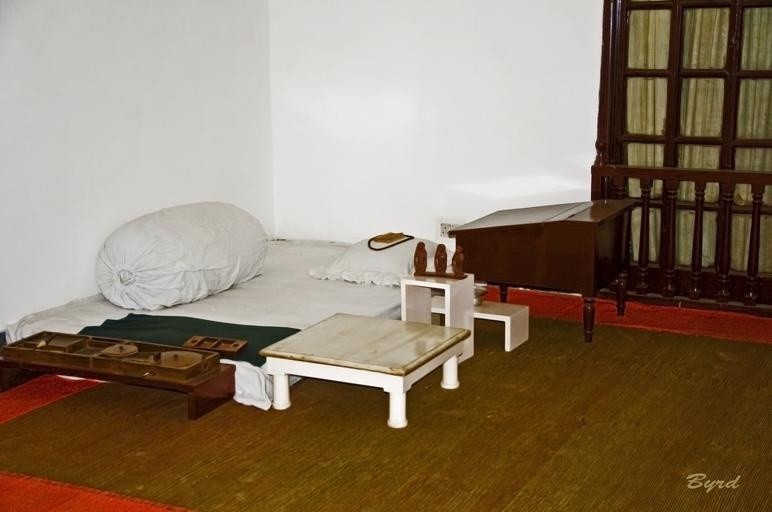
[
  {"xmin": 2, "ymin": 240, "xmax": 455, "ymax": 418},
  {"xmin": 399, "ymin": 270, "xmax": 473, "ymax": 367}
]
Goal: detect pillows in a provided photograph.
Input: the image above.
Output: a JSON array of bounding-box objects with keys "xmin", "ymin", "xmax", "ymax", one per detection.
[{"xmin": 308, "ymin": 229, "xmax": 454, "ymax": 286}]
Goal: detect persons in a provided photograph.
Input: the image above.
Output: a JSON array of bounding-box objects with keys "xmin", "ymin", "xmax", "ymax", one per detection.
[{"xmin": 414, "ymin": 241, "xmax": 465, "ymax": 276}]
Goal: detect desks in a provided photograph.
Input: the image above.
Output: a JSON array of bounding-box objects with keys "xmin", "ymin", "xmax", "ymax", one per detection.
[
  {"xmin": 2, "ymin": 331, "xmax": 235, "ymax": 419},
  {"xmin": 259, "ymin": 312, "xmax": 472, "ymax": 428}
]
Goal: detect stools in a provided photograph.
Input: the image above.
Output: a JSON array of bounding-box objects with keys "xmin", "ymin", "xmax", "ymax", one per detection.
[{"xmin": 427, "ymin": 296, "xmax": 528, "ymax": 353}]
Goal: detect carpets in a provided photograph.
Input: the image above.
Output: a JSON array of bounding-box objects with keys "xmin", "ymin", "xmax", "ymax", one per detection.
[{"xmin": 2, "ymin": 321, "xmax": 771, "ymax": 512}]
